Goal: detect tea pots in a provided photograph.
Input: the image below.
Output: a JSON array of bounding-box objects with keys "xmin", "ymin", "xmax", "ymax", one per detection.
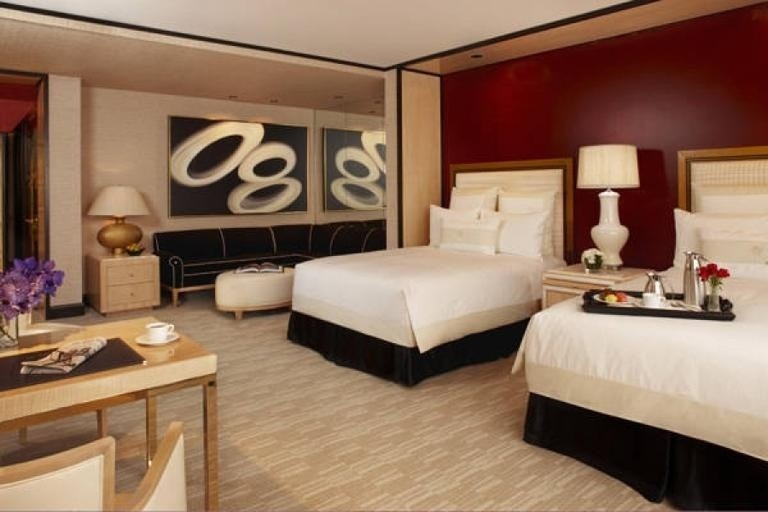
[
  {"xmin": 644, "ymin": 271, "xmax": 675, "ymax": 304},
  {"xmin": 682, "ymin": 249, "xmax": 711, "ymax": 309}
]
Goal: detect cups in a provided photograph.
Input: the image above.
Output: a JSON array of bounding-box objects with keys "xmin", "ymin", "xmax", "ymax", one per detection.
[
  {"xmin": 148, "ymin": 349, "xmax": 174, "ymax": 363},
  {"xmin": 642, "ymin": 292, "xmax": 668, "ymax": 309},
  {"xmin": 145, "ymin": 321, "xmax": 173, "ymax": 342}
]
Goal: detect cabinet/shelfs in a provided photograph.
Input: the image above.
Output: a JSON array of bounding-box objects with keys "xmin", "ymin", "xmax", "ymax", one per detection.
[{"xmin": 86, "ymin": 252, "xmax": 161, "ymax": 318}]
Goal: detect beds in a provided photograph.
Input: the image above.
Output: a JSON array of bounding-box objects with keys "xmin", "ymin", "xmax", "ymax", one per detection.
[
  {"xmin": 287, "ymin": 159, "xmax": 573, "ymax": 386},
  {"xmin": 509, "ymin": 145, "xmax": 768, "ymax": 510}
]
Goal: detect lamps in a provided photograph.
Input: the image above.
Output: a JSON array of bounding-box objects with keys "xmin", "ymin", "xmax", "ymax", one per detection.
[
  {"xmin": 85, "ymin": 184, "xmax": 151, "ymax": 256},
  {"xmin": 576, "ymin": 144, "xmax": 640, "ymax": 266}
]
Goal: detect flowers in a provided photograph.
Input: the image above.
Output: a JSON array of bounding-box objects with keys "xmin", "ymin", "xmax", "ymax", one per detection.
[
  {"xmin": 1, "ymin": 255, "xmax": 65, "ymax": 345},
  {"xmin": 694, "ymin": 260, "xmax": 730, "ymax": 286}
]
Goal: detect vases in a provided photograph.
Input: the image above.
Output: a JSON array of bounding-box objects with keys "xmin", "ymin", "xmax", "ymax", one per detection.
[
  {"xmin": 709, "ymin": 282, "xmax": 722, "ymax": 314},
  {"xmin": 0, "ymin": 313, "xmax": 21, "ymax": 350}
]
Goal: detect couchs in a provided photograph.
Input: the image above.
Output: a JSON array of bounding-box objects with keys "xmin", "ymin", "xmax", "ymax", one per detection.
[{"xmin": 154, "ymin": 217, "xmax": 386, "ymax": 309}]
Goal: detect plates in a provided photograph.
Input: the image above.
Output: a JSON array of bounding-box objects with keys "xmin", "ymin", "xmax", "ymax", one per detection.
[
  {"xmin": 134, "ymin": 333, "xmax": 179, "ymax": 345},
  {"xmin": 637, "ymin": 300, "xmax": 670, "ymax": 309},
  {"xmin": 591, "ymin": 293, "xmax": 635, "ymax": 305}
]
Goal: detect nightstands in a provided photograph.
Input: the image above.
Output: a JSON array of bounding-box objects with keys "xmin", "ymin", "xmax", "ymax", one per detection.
[{"xmin": 542, "ymin": 258, "xmax": 651, "ymax": 310}]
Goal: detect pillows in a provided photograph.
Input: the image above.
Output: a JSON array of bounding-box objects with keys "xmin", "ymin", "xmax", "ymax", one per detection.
[
  {"xmin": 479, "ymin": 210, "xmax": 549, "ymax": 264},
  {"xmin": 673, "ymin": 207, "xmax": 768, "ymax": 277},
  {"xmin": 431, "ymin": 205, "xmax": 480, "ymax": 247},
  {"xmin": 498, "ymin": 190, "xmax": 555, "ymax": 257},
  {"xmin": 448, "ymin": 187, "xmax": 501, "ymax": 211},
  {"xmin": 439, "ymin": 217, "xmax": 504, "ymax": 256}
]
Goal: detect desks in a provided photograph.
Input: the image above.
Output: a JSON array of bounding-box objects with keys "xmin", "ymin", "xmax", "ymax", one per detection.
[{"xmin": 0, "ymin": 316, "xmax": 220, "ymax": 512}]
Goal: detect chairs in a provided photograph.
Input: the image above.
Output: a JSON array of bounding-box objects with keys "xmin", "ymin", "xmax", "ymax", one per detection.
[{"xmin": 1, "ymin": 421, "xmax": 187, "ymax": 511}]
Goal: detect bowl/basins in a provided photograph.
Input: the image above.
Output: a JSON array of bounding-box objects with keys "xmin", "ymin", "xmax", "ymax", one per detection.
[{"xmin": 126, "ymin": 248, "xmax": 145, "ymax": 257}]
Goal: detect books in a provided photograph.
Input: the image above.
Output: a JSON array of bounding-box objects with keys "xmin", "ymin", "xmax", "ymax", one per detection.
[{"xmin": 231, "ymin": 262, "xmax": 284, "ymax": 275}]
